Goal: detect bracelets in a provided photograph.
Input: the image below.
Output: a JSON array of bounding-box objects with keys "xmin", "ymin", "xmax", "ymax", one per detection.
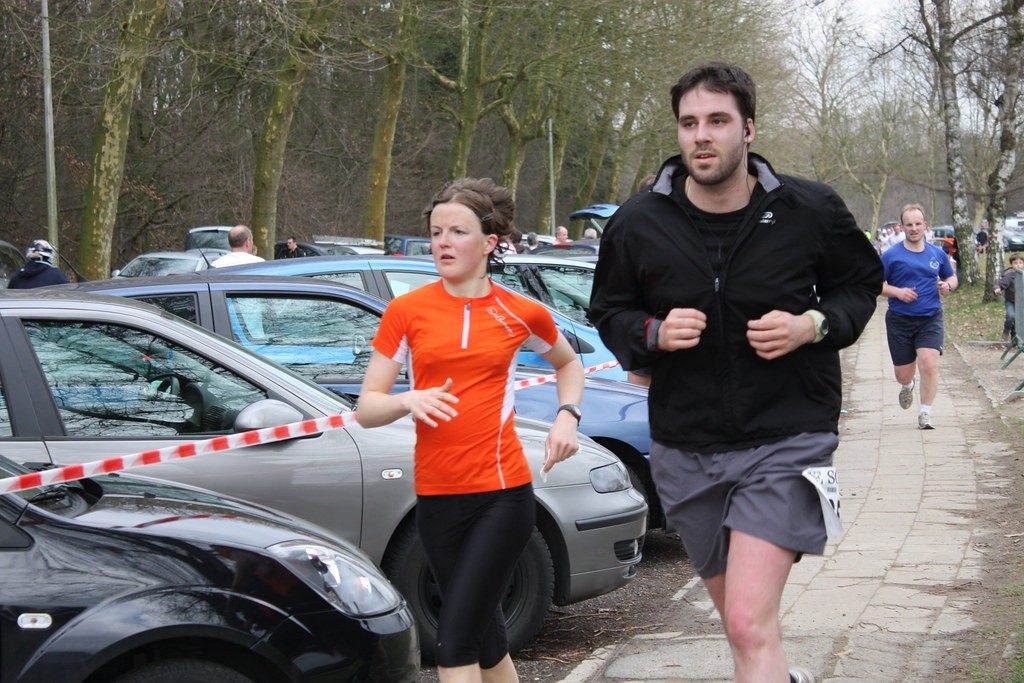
[{"xmin": 948, "ymin": 283, "xmax": 951, "ymax": 292}]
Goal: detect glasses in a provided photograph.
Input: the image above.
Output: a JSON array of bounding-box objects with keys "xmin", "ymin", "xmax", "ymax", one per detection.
[{"xmin": 286, "ymin": 242, "xmax": 292, "ymax": 244}]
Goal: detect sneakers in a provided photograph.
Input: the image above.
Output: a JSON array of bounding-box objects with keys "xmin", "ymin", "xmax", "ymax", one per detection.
[
  {"xmin": 898, "ymin": 376, "xmax": 915, "ymax": 408},
  {"xmin": 917, "ymin": 411, "xmax": 936, "ymax": 429},
  {"xmin": 1002, "ymin": 329, "xmax": 1009, "ymax": 339}
]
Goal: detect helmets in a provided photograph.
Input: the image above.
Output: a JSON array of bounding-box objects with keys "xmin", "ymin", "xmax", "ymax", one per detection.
[{"xmin": 26, "ymin": 239, "xmax": 55, "ymax": 267}]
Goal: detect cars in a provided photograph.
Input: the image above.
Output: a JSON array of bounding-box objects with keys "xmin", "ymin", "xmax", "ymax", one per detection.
[
  {"xmin": 1002, "ymin": 216, "xmax": 1024, "ymax": 253},
  {"xmin": 0, "ymin": 455, "xmax": 421, "ymax": 683},
  {"xmin": 273, "ymin": 203, "xmax": 621, "ymax": 330},
  {"xmin": 108, "ymin": 227, "xmax": 235, "ymax": 280},
  {"xmin": 931, "ymin": 226, "xmax": 958, "ymax": 258},
  {"xmin": 0, "ymin": 276, "xmax": 667, "ymax": 532},
  {"xmin": 186, "ymin": 253, "xmax": 629, "ymax": 384},
  {"xmin": 0, "ymin": 287, "xmax": 650, "ymax": 665}
]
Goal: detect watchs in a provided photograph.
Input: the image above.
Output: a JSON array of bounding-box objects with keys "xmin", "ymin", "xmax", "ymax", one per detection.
[
  {"xmin": 558, "ymin": 404, "xmax": 582, "ymax": 426},
  {"xmin": 803, "ymin": 309, "xmax": 829, "ymax": 343}
]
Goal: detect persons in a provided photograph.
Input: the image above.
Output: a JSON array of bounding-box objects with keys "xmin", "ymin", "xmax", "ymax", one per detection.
[
  {"xmin": 977, "ymin": 228, "xmax": 988, "ymax": 253},
  {"xmin": 880, "ymin": 206, "xmax": 959, "ymax": 429},
  {"xmin": 212, "ymin": 225, "xmax": 265, "ymax": 268},
  {"xmin": 504, "ymin": 232, "xmax": 538, "ymax": 254},
  {"xmin": 356, "ymin": 178, "xmax": 585, "ymax": 683},
  {"xmin": 926, "ymin": 226, "xmax": 936, "ymax": 244},
  {"xmin": 865, "ymin": 224, "xmax": 905, "ymax": 254},
  {"xmin": 9, "ymin": 240, "xmax": 68, "ymax": 290},
  {"xmin": 995, "ymin": 254, "xmax": 1024, "ymax": 341},
  {"xmin": 554, "ymin": 226, "xmax": 567, "ymax": 245},
  {"xmin": 590, "ymin": 66, "xmax": 888, "ymax": 683},
  {"xmin": 585, "ymin": 229, "xmax": 596, "ymax": 240},
  {"xmin": 281, "ymin": 236, "xmax": 305, "ymax": 259}
]
